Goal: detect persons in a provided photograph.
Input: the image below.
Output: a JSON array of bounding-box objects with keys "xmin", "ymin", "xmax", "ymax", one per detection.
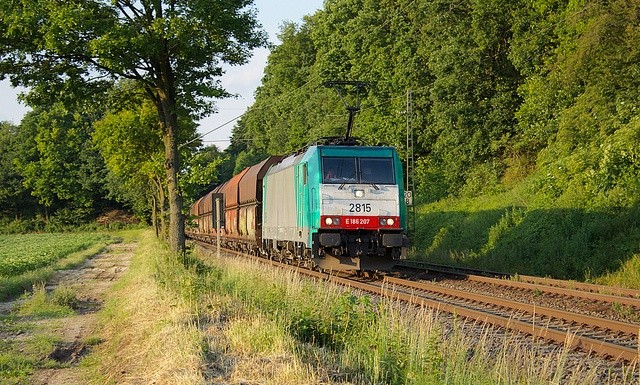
[{"xmin": 326, "ymin": 167, "xmax": 336, "ymax": 179}]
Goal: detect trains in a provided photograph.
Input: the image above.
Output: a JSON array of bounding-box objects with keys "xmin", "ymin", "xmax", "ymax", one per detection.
[{"xmin": 190, "ymin": 137, "xmax": 406, "ymax": 281}]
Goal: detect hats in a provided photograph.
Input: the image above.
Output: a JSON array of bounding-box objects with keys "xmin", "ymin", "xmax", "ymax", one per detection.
[{"xmin": 328, "ymin": 167, "xmax": 337, "ymax": 175}]
